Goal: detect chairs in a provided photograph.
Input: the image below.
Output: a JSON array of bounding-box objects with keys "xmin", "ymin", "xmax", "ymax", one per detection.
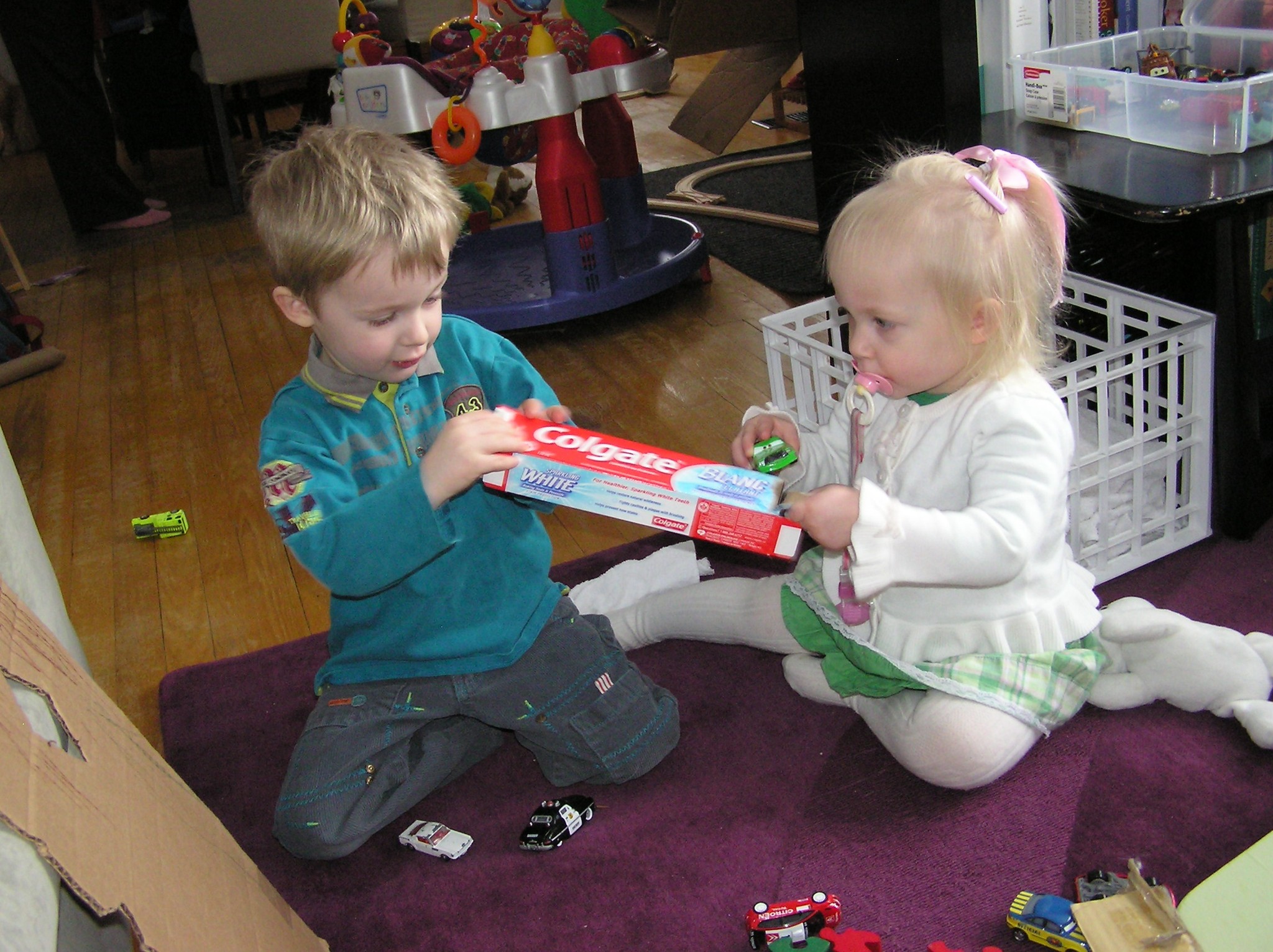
[{"xmin": 187, "ymin": 0, "xmax": 341, "ymax": 214}]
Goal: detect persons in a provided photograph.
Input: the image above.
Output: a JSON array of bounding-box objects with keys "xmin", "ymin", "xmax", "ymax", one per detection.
[
  {"xmin": 0, "ymin": 0, "xmax": 172, "ymax": 232},
  {"xmin": 246, "ymin": 123, "xmax": 679, "ymax": 861},
  {"xmin": 608, "ymin": 145, "xmax": 1110, "ymax": 791}
]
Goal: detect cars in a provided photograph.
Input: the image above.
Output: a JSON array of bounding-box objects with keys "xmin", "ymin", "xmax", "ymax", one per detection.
[
  {"xmin": 745, "ymin": 891, "xmax": 842, "ymax": 950},
  {"xmin": 519, "ymin": 794, "xmax": 596, "ymax": 852},
  {"xmin": 1006, "ymin": 890, "xmax": 1093, "ymax": 952},
  {"xmin": 130, "ymin": 509, "xmax": 189, "ymax": 540},
  {"xmin": 1074, "ymin": 868, "xmax": 1176, "ymax": 909},
  {"xmin": 398, "ymin": 819, "xmax": 474, "ymax": 861}
]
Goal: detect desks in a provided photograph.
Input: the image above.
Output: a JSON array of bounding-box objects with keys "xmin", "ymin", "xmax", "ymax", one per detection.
[{"xmin": 882, "ymin": 111, "xmax": 1273, "ymax": 542}]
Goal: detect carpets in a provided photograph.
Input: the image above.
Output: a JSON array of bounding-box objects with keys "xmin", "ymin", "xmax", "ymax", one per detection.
[
  {"xmin": 644, "ymin": 136, "xmax": 823, "ymax": 294},
  {"xmin": 161, "ymin": 528, "xmax": 1273, "ymax": 952}
]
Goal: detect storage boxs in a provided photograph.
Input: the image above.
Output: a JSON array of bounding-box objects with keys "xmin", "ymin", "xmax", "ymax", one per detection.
[
  {"xmin": 1006, "ymin": 0, "xmax": 1273, "ymax": 155},
  {"xmin": 484, "ymin": 405, "xmax": 803, "ymax": 559},
  {"xmin": 757, "ymin": 271, "xmax": 1215, "ymax": 585}
]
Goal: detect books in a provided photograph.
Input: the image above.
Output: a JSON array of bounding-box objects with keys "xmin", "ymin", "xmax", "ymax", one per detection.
[{"xmin": 1082, "ymin": 592, "xmax": 1273, "ymax": 750}]
